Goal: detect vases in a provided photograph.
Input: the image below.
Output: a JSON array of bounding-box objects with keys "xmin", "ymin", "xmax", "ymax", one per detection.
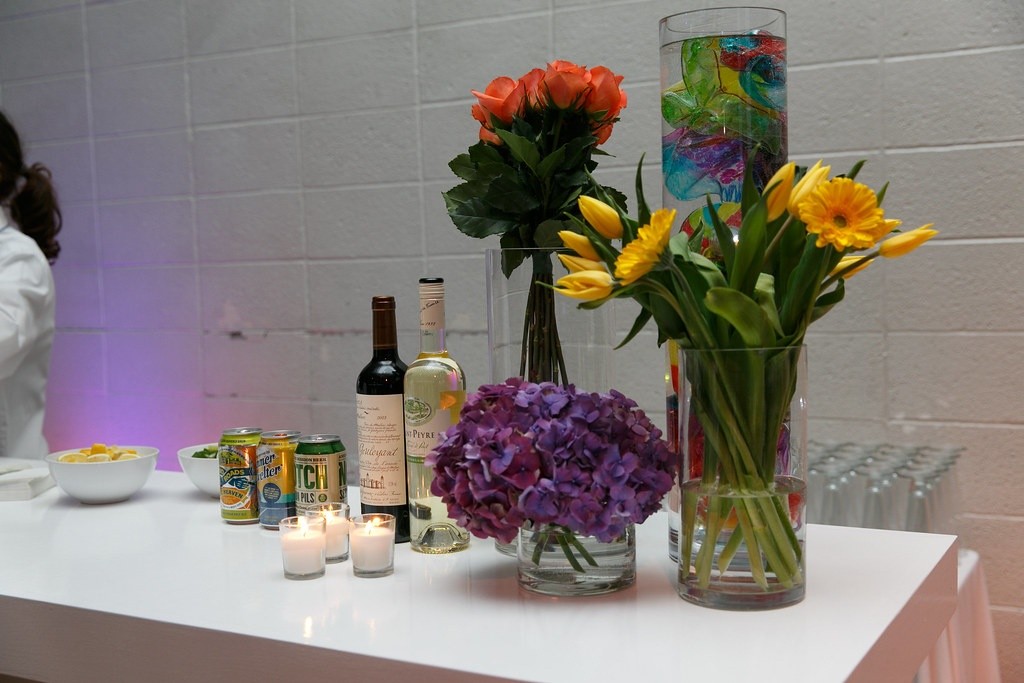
[
  {"xmin": 674, "ymin": 344, "xmax": 808, "ymax": 615},
  {"xmin": 659, "ymin": 6, "xmax": 788, "ymax": 565},
  {"xmin": 488, "ymin": 521, "xmax": 629, "ymax": 560},
  {"xmin": 517, "ymin": 521, "xmax": 636, "ymax": 595}
]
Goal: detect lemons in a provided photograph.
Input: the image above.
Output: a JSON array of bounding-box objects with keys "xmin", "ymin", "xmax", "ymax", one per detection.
[{"xmin": 58, "ymin": 443, "xmax": 142, "ymax": 462}]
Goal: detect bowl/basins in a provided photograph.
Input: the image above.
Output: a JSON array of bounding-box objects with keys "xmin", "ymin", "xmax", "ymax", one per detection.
[
  {"xmin": 175, "ymin": 442, "xmax": 221, "ymax": 500},
  {"xmin": 45, "ymin": 446, "xmax": 159, "ymax": 503}
]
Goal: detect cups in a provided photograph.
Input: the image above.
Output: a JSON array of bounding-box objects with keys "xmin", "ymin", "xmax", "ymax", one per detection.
[
  {"xmin": 306, "ymin": 503, "xmax": 350, "ymax": 564},
  {"xmin": 347, "ymin": 513, "xmax": 396, "ymax": 577},
  {"xmin": 277, "ymin": 515, "xmax": 327, "ymax": 580}
]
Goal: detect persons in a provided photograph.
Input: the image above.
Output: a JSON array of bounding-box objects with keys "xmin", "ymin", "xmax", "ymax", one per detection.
[{"xmin": 0, "ymin": 111, "xmax": 63, "ymax": 459}]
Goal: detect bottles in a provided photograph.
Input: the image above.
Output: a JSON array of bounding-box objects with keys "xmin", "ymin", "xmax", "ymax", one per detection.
[
  {"xmin": 404, "ymin": 277, "xmax": 470, "ymax": 555},
  {"xmin": 355, "ymin": 295, "xmax": 411, "ymax": 544}
]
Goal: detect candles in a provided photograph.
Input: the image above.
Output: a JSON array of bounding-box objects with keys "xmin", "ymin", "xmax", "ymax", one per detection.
[
  {"xmin": 348, "ymin": 514, "xmax": 396, "ymax": 578},
  {"xmin": 306, "ymin": 502, "xmax": 350, "ymax": 564},
  {"xmin": 280, "ymin": 515, "xmax": 326, "ymax": 580}
]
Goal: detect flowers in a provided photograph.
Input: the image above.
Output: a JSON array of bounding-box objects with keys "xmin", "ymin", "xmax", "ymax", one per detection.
[
  {"xmin": 532, "ymin": 154, "xmax": 941, "ymax": 593},
  {"xmin": 426, "ymin": 379, "xmax": 671, "ymax": 546},
  {"xmin": 439, "ymin": 61, "xmax": 632, "ymax": 387}
]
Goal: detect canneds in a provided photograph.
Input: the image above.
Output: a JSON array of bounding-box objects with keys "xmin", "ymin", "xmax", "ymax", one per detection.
[{"xmin": 218, "ymin": 427, "xmax": 348, "ymax": 531}]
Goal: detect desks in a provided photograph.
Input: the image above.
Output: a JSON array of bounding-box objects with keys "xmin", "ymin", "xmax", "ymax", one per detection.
[{"xmin": 0, "ymin": 454, "xmax": 957, "ymax": 683}]
